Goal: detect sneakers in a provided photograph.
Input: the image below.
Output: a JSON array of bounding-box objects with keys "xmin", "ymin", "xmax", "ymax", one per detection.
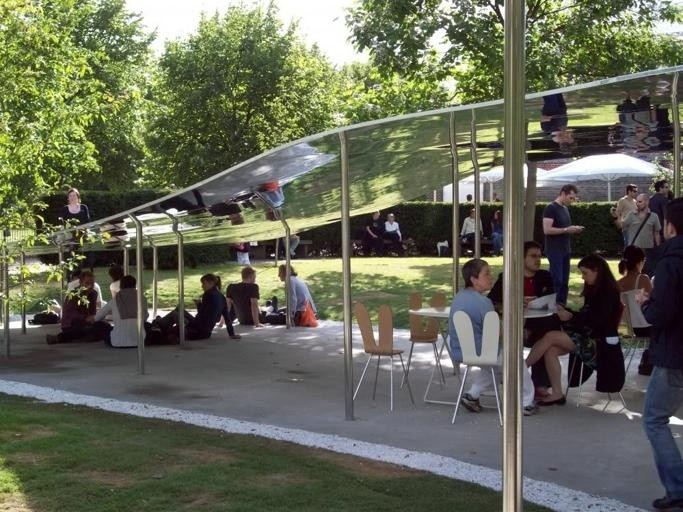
[{"xmin": 460, "ymin": 394, "xmax": 483, "ymax": 413}]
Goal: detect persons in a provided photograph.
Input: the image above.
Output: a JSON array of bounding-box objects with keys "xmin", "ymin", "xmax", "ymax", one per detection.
[
  {"xmin": 486, "ymin": 240, "xmax": 557, "ymax": 399},
  {"xmin": 634, "ymin": 197, "xmax": 683, "ymax": 512},
  {"xmin": 538, "ymin": 94, "xmax": 673, "ymax": 154},
  {"xmin": 281, "ymin": 205, "xmax": 505, "ymax": 263},
  {"xmin": 542, "ymin": 183, "xmax": 585, "ymax": 310},
  {"xmin": 526, "ymin": 254, "xmax": 624, "ymax": 405},
  {"xmin": 205, "ymin": 181, "xmax": 291, "ymax": 223},
  {"xmin": 448, "ymin": 258, "xmax": 539, "ymax": 416},
  {"xmin": 235, "ymin": 240, "xmax": 255, "ymax": 265},
  {"xmin": 60, "ymin": 183, "xmax": 91, "ymax": 227},
  {"xmin": 608, "ymin": 182, "xmax": 682, "ymax": 344},
  {"xmin": 44, "ymin": 260, "xmax": 321, "ymax": 349}
]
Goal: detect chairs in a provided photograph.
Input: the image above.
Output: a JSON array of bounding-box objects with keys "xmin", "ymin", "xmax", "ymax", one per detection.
[
  {"xmin": 620, "ymin": 292, "xmax": 650, "ymax": 376},
  {"xmin": 352, "ymin": 304, "xmax": 415, "ymax": 412},
  {"xmin": 399, "ymin": 290, "xmax": 460, "ymax": 389},
  {"xmin": 451, "ymin": 310, "xmax": 505, "ymax": 428},
  {"xmin": 564, "ymin": 300, "xmax": 629, "ymax": 409}
]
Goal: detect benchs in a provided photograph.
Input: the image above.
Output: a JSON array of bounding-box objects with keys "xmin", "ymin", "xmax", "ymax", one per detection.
[
  {"xmin": 248, "ymin": 239, "xmax": 314, "ymax": 259},
  {"xmin": 351, "ymin": 238, "xmax": 416, "ymax": 257},
  {"xmin": 461, "ymin": 239, "xmax": 495, "ymax": 257}
]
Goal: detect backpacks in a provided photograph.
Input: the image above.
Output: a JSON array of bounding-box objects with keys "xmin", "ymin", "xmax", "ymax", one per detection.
[{"xmin": 28, "ymin": 313, "xmax": 58, "ymax": 324}]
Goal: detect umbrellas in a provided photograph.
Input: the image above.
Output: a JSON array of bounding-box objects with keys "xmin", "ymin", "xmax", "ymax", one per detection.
[
  {"xmin": 547, "ymin": 155, "xmax": 674, "ymax": 199},
  {"xmin": 467, "ymin": 161, "xmax": 549, "ymax": 185}
]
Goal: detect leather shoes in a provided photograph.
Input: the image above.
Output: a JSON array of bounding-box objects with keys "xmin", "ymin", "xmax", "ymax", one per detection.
[
  {"xmin": 653, "ymin": 496, "xmax": 683, "ymax": 508},
  {"xmin": 523, "ymin": 390, "xmax": 566, "ymax": 416}
]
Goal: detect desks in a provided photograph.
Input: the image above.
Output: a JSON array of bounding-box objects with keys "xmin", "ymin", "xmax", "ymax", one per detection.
[{"xmin": 409, "ymin": 308, "xmax": 553, "ymax": 410}]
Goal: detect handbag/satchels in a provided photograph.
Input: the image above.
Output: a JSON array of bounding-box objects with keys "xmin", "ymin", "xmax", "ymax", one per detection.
[{"xmin": 621, "ymin": 274, "xmax": 652, "ymax": 329}]
